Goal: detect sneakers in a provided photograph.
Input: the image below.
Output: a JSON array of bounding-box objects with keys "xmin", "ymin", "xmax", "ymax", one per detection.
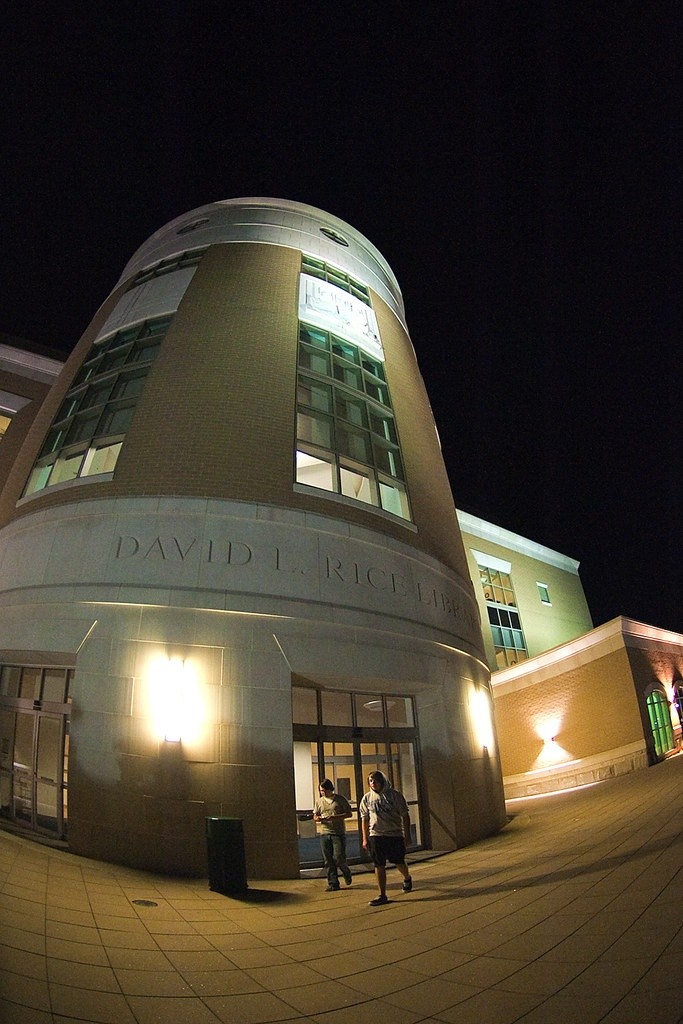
[
  {"xmin": 341, "ymin": 871, "xmax": 353, "ymax": 885},
  {"xmin": 368, "ymin": 893, "xmax": 389, "ymax": 906},
  {"xmin": 325, "ymin": 882, "xmax": 340, "ymax": 892},
  {"xmin": 402, "ymin": 876, "xmax": 413, "ymax": 893}
]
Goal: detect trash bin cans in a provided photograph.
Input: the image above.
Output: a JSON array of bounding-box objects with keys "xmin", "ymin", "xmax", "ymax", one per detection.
[{"xmin": 205, "ymin": 816, "xmax": 249, "ymax": 893}]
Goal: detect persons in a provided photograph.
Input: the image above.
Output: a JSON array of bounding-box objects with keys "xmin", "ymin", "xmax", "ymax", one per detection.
[
  {"xmin": 359, "ymin": 771, "xmax": 412, "ymax": 907},
  {"xmin": 313, "ymin": 779, "xmax": 353, "ymax": 892}
]
